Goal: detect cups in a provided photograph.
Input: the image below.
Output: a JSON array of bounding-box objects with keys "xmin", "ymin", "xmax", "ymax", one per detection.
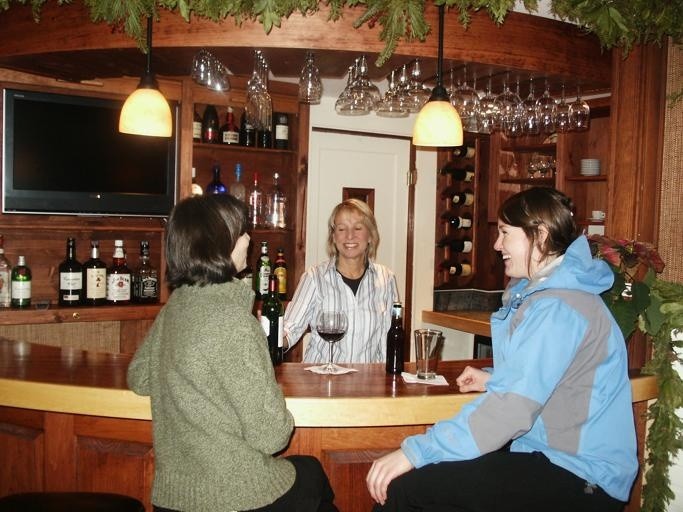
[
  {"xmin": 591, "ymin": 210, "xmax": 605, "ymax": 219},
  {"xmin": 415, "ymin": 330, "xmax": 444, "ymax": 380}
]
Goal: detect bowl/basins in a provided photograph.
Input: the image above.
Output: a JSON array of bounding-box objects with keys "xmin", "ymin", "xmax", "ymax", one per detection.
[{"xmin": 578, "ymin": 157, "xmax": 598, "ymax": 175}]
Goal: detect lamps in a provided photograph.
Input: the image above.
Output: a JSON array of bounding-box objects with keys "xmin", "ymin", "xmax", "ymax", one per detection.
[
  {"xmin": 410, "ymin": 3, "xmax": 468, "ymax": 148},
  {"xmin": 116, "ymin": 13, "xmax": 174, "ymax": 139}
]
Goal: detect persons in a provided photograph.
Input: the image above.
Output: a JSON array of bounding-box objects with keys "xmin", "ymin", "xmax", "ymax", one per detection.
[
  {"xmin": 256, "ymin": 196, "xmax": 399, "ymax": 364},
  {"xmin": 126, "ymin": 194, "xmax": 339, "ymax": 510},
  {"xmin": 364, "ymin": 184, "xmax": 640, "ymax": 510}
]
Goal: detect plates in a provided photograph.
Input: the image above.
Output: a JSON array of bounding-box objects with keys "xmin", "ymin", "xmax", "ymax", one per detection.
[{"xmin": 586, "ymin": 218, "xmax": 604, "ymax": 222}]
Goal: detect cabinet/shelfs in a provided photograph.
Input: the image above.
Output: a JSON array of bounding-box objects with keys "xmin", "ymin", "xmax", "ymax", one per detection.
[
  {"xmin": 180, "ymin": 77, "xmax": 311, "ymax": 317},
  {"xmin": 439, "ymin": 108, "xmax": 609, "ymax": 291}
]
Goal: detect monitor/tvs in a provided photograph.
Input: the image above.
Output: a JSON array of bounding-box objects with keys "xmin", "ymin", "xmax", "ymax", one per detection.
[{"xmin": 1, "ymin": 87, "xmax": 179, "ymax": 217}]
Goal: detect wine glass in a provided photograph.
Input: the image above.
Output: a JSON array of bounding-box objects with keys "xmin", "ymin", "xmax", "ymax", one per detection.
[
  {"xmin": 539, "ymin": 154, "xmax": 549, "ymax": 178},
  {"xmin": 314, "ymin": 313, "xmax": 347, "ymax": 373},
  {"xmin": 527, "ymin": 158, "xmax": 536, "ymax": 179}
]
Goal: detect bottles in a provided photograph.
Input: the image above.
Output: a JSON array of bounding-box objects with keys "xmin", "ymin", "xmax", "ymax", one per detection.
[
  {"xmin": 437, "ymin": 137, "xmax": 475, "ymax": 282},
  {"xmin": 273, "ymin": 248, "xmax": 289, "ymax": 297},
  {"xmin": 255, "ymin": 242, "xmax": 270, "ymax": 295},
  {"xmin": 84, "ymin": 241, "xmax": 105, "ymax": 304},
  {"xmin": 192, "ymin": 103, "xmax": 289, "ymax": 146},
  {"xmin": 0, "ymin": 235, "xmax": 11, "ymax": 308},
  {"xmin": 59, "ymin": 238, "xmax": 82, "ymax": 302},
  {"xmin": 248, "ymin": 173, "xmax": 262, "ymax": 228},
  {"xmin": 190, "ymin": 160, "xmax": 246, "ymax": 221},
  {"xmin": 261, "ymin": 276, "xmax": 283, "ymax": 362},
  {"xmin": 134, "ymin": 241, "xmax": 158, "ymax": 302},
  {"xmin": 265, "ymin": 172, "xmax": 286, "ymax": 228},
  {"xmin": 12, "ymin": 256, "xmax": 30, "ymax": 305},
  {"xmin": 107, "ymin": 239, "xmax": 131, "ymax": 303},
  {"xmin": 386, "ymin": 303, "xmax": 405, "ymax": 373}
]
